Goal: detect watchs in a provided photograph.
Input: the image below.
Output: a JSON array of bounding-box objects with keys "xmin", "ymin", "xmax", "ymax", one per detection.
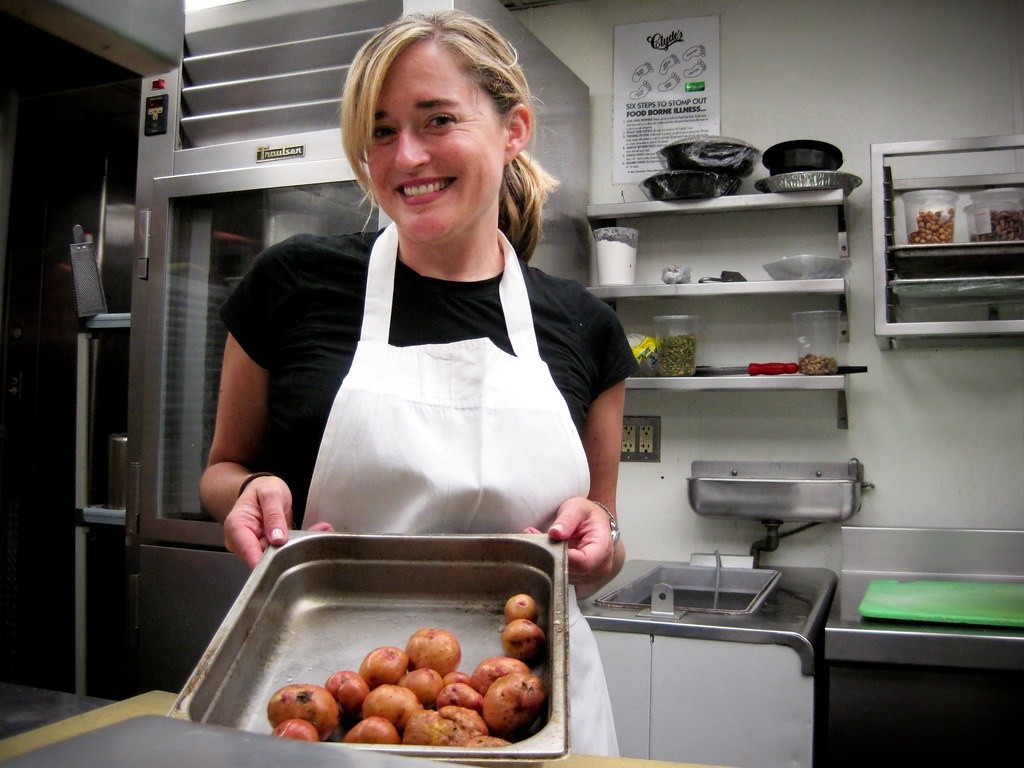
[{"xmin": 593, "ymin": 500, "xmax": 620, "ymax": 544}]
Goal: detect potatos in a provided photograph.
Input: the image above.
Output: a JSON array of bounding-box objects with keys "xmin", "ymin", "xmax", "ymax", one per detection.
[{"xmin": 266, "ymin": 593, "xmax": 547, "ymax": 747}]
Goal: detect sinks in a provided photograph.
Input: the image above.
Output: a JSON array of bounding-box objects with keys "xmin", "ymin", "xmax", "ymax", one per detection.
[{"xmin": 686, "ymin": 477, "xmax": 862, "ymax": 523}]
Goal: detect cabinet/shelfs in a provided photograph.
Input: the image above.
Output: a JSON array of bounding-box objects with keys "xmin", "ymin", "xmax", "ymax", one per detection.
[
  {"xmin": 587, "ymin": 630, "xmax": 814, "ymax": 768},
  {"xmin": 585, "ymin": 189, "xmax": 849, "ymax": 430},
  {"xmin": 871, "ymin": 133, "xmax": 1024, "ymax": 351}
]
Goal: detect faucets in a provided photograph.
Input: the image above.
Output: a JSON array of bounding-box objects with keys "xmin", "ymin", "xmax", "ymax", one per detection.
[{"xmin": 847, "ymin": 457, "xmax": 859, "ymax": 476}]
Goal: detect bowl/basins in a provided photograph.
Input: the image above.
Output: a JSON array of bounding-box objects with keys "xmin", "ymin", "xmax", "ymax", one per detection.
[
  {"xmin": 762, "ymin": 254, "xmax": 853, "ymax": 280},
  {"xmin": 642, "ymin": 138, "xmax": 862, "ymax": 199}
]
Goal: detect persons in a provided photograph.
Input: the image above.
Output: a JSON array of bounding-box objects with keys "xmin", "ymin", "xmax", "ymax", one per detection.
[{"xmin": 198, "ymin": 6, "xmax": 624, "ymax": 760}]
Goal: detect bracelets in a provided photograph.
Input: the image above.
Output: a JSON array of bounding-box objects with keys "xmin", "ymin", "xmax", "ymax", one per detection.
[{"xmin": 236, "ymin": 472, "xmax": 275, "ymax": 498}]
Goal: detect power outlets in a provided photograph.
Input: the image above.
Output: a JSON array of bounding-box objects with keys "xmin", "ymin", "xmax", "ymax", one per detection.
[{"xmin": 620, "ymin": 416, "xmax": 661, "ymax": 462}]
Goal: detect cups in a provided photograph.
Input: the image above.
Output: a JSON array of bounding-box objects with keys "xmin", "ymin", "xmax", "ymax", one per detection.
[
  {"xmin": 792, "ymin": 309, "xmax": 842, "ymax": 374},
  {"xmin": 653, "ymin": 314, "xmax": 697, "ymax": 377},
  {"xmin": 901, "ymin": 189, "xmax": 959, "ymax": 243},
  {"xmin": 964, "ymin": 204, "xmax": 980, "ymax": 242},
  {"xmin": 968, "ymin": 186, "xmax": 1024, "ymax": 241},
  {"xmin": 593, "ymin": 226, "xmax": 640, "ymax": 285}
]
